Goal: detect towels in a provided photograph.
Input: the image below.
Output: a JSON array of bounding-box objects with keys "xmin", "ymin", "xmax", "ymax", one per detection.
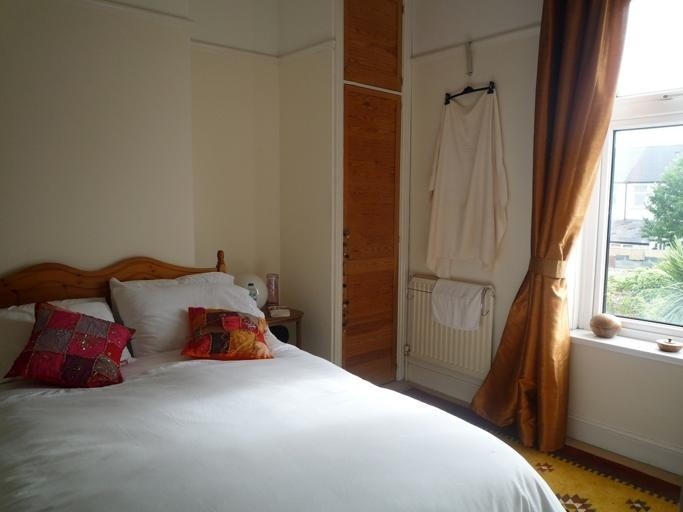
[
  {"xmin": 430, "ymin": 278, "xmax": 493, "ymax": 331},
  {"xmin": 425, "ymin": 90, "xmax": 513, "ymax": 278}
]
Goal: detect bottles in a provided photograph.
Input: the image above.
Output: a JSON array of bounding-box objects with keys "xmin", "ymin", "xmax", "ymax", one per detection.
[
  {"xmin": 246, "ymin": 283, "xmax": 256, "ymax": 301},
  {"xmin": 265, "ymin": 273, "xmax": 279, "ymax": 307}
]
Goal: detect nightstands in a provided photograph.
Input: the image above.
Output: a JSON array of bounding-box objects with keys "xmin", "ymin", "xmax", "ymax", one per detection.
[{"xmin": 262, "ymin": 306, "xmax": 304, "ymax": 347}]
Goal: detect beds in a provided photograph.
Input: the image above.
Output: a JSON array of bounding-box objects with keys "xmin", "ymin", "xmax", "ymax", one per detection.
[{"xmin": 0, "ymin": 249, "xmax": 563, "ymax": 512}]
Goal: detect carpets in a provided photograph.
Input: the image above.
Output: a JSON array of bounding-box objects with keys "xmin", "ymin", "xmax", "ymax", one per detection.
[{"xmin": 380, "ymin": 379, "xmax": 683, "ymax": 512}]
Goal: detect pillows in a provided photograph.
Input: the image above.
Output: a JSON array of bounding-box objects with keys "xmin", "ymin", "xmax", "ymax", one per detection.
[{"xmin": 0, "ymin": 271, "xmax": 274, "ymax": 389}]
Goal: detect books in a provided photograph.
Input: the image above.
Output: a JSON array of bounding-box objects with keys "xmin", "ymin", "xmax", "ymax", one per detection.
[{"xmin": 268, "ymin": 305, "xmax": 291, "ymax": 317}]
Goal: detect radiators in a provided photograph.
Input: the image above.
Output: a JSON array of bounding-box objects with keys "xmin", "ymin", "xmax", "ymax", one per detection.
[{"xmin": 408, "ymin": 275, "xmax": 494, "ymax": 384}]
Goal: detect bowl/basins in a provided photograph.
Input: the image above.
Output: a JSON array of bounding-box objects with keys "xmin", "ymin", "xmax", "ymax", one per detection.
[{"xmin": 656, "ymin": 338, "xmax": 682, "ymax": 352}]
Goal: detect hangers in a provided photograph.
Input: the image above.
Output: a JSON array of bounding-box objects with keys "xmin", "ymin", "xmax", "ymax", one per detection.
[{"xmin": 444, "ymin": 73, "xmax": 495, "ymax": 106}]
